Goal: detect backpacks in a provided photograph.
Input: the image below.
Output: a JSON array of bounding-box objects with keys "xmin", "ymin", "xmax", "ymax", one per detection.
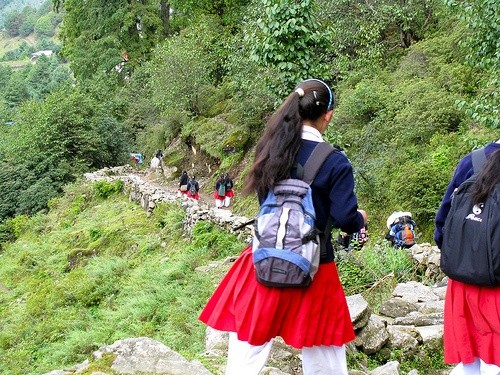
[
  {"xmin": 252, "ymin": 141, "xmax": 341, "ymax": 288},
  {"xmin": 440, "ymin": 146, "xmax": 500, "ymax": 287},
  {"xmin": 394, "ymin": 221, "xmax": 415, "ymax": 249},
  {"xmin": 189, "ymin": 181, "xmax": 196, "ymax": 195},
  {"xmin": 351, "ymin": 223, "xmax": 368, "ymax": 244},
  {"xmin": 226, "ymin": 180, "xmax": 232, "ymax": 191},
  {"xmin": 218, "ymin": 181, "xmax": 226, "ymax": 196}
]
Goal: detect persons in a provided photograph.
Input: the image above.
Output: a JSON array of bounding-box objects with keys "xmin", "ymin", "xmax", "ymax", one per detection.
[
  {"xmin": 338, "ymin": 208, "xmax": 418, "ymax": 250},
  {"xmin": 434, "ymin": 138, "xmax": 500, "ymax": 375},
  {"xmin": 131, "ymin": 148, "xmax": 235, "ymax": 210},
  {"xmin": 198, "ymin": 79, "xmax": 367, "ymax": 375}
]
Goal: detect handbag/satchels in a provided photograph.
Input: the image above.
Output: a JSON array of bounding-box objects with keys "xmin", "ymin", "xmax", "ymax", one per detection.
[{"xmin": 181, "ymin": 185, "xmax": 188, "ymax": 192}]
